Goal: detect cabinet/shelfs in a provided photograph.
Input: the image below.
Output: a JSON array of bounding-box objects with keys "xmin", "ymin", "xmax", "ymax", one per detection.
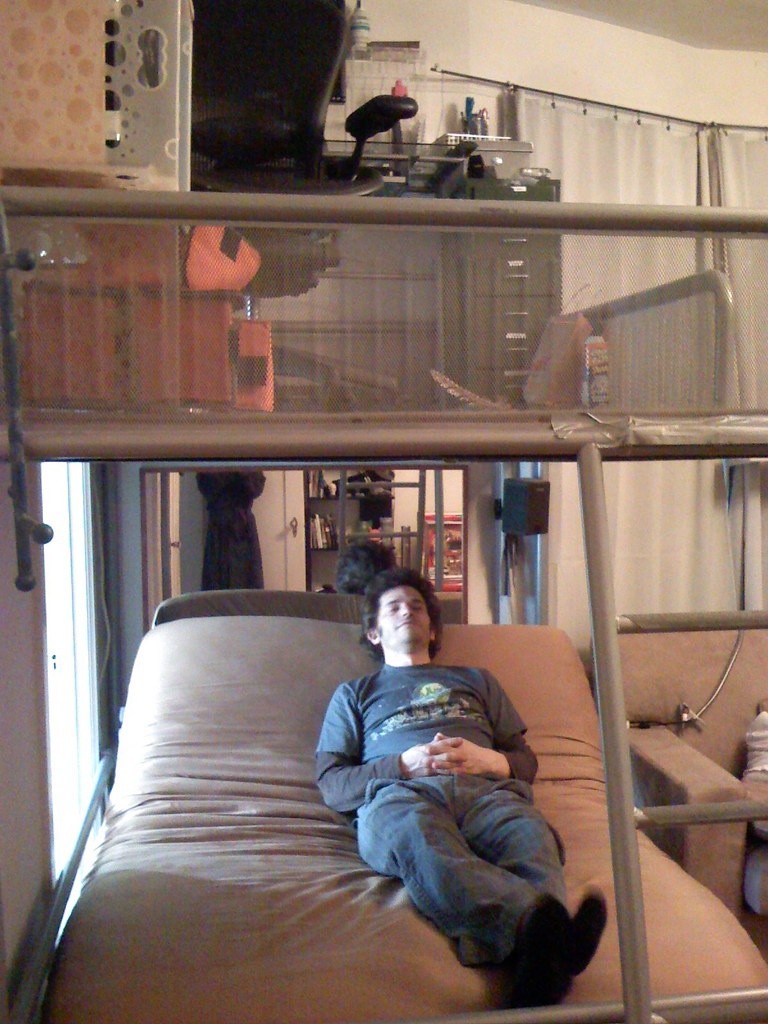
[
  {"xmin": 455, "ymin": 176, "xmax": 560, "ymax": 410},
  {"xmin": 303, "ymin": 469, "xmax": 426, "ymax": 592}
]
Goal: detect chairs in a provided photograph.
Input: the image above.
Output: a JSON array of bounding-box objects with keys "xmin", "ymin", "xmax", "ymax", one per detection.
[{"xmin": 191, "ymin": 0, "xmax": 417, "ymax": 414}]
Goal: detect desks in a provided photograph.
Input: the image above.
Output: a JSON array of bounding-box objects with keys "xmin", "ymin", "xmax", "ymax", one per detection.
[{"xmin": 234, "ymin": 139, "xmax": 465, "ymax": 414}]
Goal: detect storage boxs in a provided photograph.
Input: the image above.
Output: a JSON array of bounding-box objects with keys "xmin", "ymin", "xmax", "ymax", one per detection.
[{"xmin": 466, "ymin": 140, "xmax": 533, "ymax": 179}]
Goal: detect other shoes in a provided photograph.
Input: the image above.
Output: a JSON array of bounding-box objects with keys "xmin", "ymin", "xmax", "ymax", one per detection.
[
  {"xmin": 508, "ymin": 894, "xmax": 572, "ymax": 1010},
  {"xmin": 567, "ymin": 881, "xmax": 607, "ymax": 976}
]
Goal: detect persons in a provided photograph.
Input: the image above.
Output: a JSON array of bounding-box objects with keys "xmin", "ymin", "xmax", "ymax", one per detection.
[
  {"xmin": 336, "ymin": 542, "xmax": 395, "ymax": 594},
  {"xmin": 317, "ymin": 567, "xmax": 606, "ymax": 1012}
]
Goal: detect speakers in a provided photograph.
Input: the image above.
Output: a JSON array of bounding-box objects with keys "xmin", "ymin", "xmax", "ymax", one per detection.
[{"xmin": 503, "ymin": 477, "xmax": 550, "ymax": 535}]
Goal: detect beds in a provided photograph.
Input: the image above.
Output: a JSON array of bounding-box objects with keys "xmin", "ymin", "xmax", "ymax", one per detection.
[
  {"xmin": 38, "ymin": 616, "xmax": 768, "ymax": 1024},
  {"xmin": 151, "ymin": 588, "xmax": 461, "ymax": 626}
]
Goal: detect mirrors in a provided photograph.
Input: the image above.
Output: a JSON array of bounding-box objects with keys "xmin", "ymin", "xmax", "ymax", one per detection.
[{"xmin": 138, "ymin": 464, "xmax": 470, "ymax": 634}]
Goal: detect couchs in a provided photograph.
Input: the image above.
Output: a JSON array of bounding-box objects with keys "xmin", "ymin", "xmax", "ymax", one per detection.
[
  {"xmin": 587, "ymin": 634, "xmax": 768, "ymax": 947},
  {"xmin": 1, "ymin": 166, "xmax": 273, "ymax": 414}
]
